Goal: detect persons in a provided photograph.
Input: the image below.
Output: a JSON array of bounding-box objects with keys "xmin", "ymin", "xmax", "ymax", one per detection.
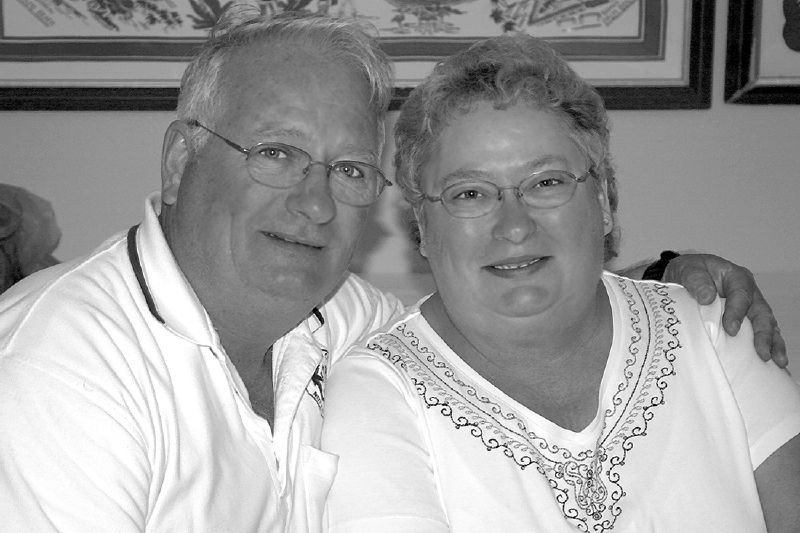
[
  {"xmin": 0, "ymin": 0, "xmax": 789, "ymax": 533},
  {"xmin": 319, "ymin": 33, "xmax": 800, "ymax": 533}
]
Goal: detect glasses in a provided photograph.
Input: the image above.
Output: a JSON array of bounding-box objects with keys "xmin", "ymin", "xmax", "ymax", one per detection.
[
  {"xmin": 187, "ymin": 120, "xmax": 393, "ymax": 209},
  {"xmin": 420, "ymin": 162, "xmax": 597, "ymax": 219}
]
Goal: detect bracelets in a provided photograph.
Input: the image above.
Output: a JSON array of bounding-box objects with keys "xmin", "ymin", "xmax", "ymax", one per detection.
[{"xmin": 641, "ymin": 250, "xmax": 681, "ymax": 282}]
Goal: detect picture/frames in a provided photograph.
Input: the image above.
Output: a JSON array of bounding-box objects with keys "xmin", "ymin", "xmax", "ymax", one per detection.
[{"xmin": 0, "ymin": 0, "xmax": 715, "ymax": 111}]
